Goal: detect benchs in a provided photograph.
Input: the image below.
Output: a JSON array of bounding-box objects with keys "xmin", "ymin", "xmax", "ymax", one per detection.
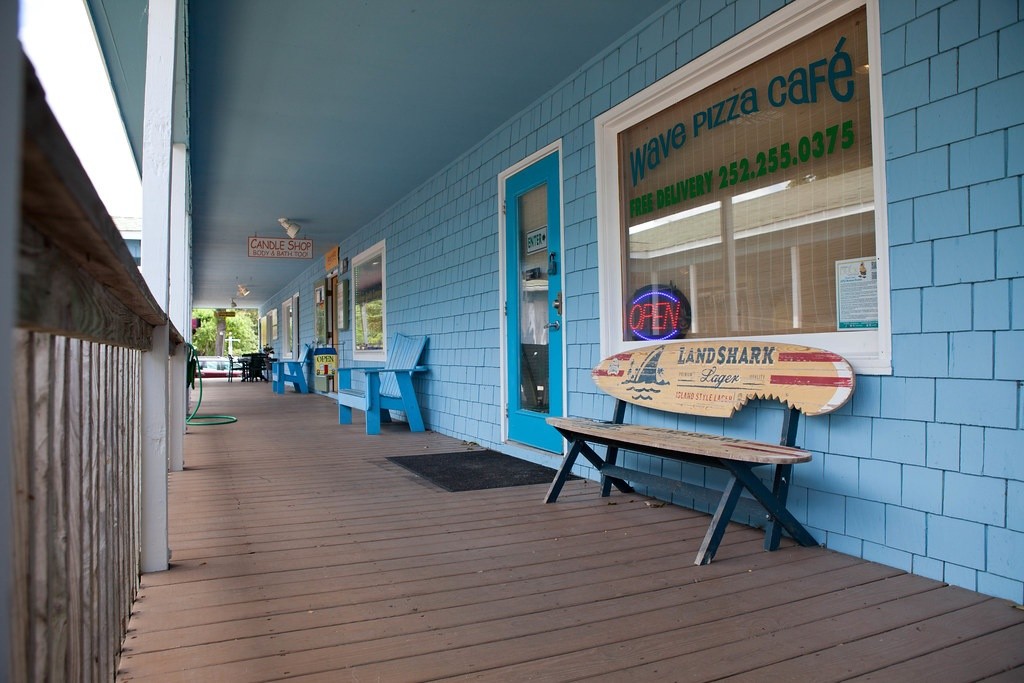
[
  {"xmin": 270, "ymin": 343, "xmax": 310, "ymax": 394},
  {"xmin": 339, "ymin": 332, "xmax": 430, "ymax": 434},
  {"xmin": 543, "ymin": 340, "xmax": 855, "ymax": 567}
]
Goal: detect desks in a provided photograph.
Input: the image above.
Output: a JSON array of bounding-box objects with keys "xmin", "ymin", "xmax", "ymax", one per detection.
[{"xmin": 241, "ymin": 352, "xmax": 268, "ymax": 383}]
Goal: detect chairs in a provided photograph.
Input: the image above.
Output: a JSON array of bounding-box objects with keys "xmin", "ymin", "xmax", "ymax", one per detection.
[
  {"xmin": 228, "ymin": 354, "xmax": 244, "ymax": 382},
  {"xmin": 238, "ymin": 358, "xmax": 250, "ymax": 382}
]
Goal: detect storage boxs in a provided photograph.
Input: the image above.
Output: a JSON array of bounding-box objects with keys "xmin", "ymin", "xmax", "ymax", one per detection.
[{"xmin": 315, "ymin": 375, "xmax": 334, "ymax": 393}]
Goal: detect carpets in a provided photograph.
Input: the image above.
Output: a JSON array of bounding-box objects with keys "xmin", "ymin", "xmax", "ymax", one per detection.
[{"xmin": 385, "ymin": 450, "xmax": 586, "ymax": 492}]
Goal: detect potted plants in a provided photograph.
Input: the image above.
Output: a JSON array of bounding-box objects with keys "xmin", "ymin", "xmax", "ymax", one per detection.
[{"xmin": 262, "ymin": 344, "xmax": 274, "ymax": 355}]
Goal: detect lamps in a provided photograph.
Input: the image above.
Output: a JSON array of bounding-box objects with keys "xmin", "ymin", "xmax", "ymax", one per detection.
[
  {"xmin": 280, "ymin": 219, "xmax": 300, "ymax": 239},
  {"xmin": 238, "ymin": 286, "xmax": 250, "ymax": 296},
  {"xmin": 231, "ymin": 299, "xmax": 236, "ymax": 307}
]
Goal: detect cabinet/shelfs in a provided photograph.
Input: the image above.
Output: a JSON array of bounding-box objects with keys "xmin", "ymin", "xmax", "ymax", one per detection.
[{"xmin": 307, "ymin": 348, "xmax": 338, "ymax": 391}]
